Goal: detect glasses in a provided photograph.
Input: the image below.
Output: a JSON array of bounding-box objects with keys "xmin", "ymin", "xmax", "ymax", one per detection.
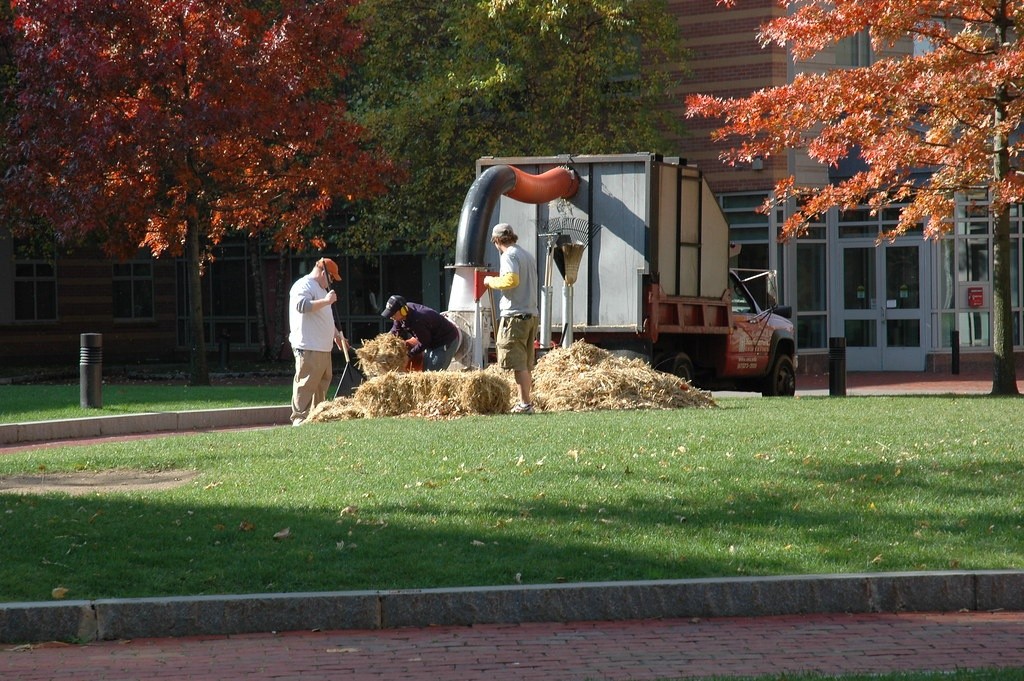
[{"xmin": 328, "ymin": 273, "xmax": 335, "ymax": 281}]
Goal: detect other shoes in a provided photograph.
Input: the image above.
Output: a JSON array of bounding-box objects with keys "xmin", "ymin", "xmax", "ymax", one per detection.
[{"xmin": 292, "ymin": 417, "xmax": 306, "ymax": 426}]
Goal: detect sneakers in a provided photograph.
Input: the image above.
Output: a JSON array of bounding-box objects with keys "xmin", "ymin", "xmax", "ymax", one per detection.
[{"xmin": 509, "ymin": 403, "xmax": 536, "ymax": 414}]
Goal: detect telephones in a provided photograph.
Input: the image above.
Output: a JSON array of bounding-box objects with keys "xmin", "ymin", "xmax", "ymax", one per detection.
[{"xmin": 968, "ymin": 287, "xmax": 983, "ymax": 306}]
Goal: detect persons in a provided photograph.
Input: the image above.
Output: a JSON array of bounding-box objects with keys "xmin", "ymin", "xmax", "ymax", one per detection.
[
  {"xmin": 484, "ymin": 223, "xmax": 538, "ymax": 413},
  {"xmin": 289, "ymin": 258, "xmax": 350, "ymax": 426},
  {"xmin": 381, "ymin": 295, "xmax": 459, "ymax": 371}
]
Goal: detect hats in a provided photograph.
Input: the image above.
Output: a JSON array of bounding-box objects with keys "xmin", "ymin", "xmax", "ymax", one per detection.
[
  {"xmin": 492, "ymin": 223, "xmax": 515, "ymax": 237},
  {"xmin": 381, "ymin": 295, "xmax": 406, "ymax": 317},
  {"xmin": 315, "ymin": 258, "xmax": 342, "ymax": 281}
]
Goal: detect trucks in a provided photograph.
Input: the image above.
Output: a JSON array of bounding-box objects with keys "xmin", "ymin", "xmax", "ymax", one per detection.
[{"xmin": 404, "ymin": 150, "xmax": 797, "ymax": 398}]
